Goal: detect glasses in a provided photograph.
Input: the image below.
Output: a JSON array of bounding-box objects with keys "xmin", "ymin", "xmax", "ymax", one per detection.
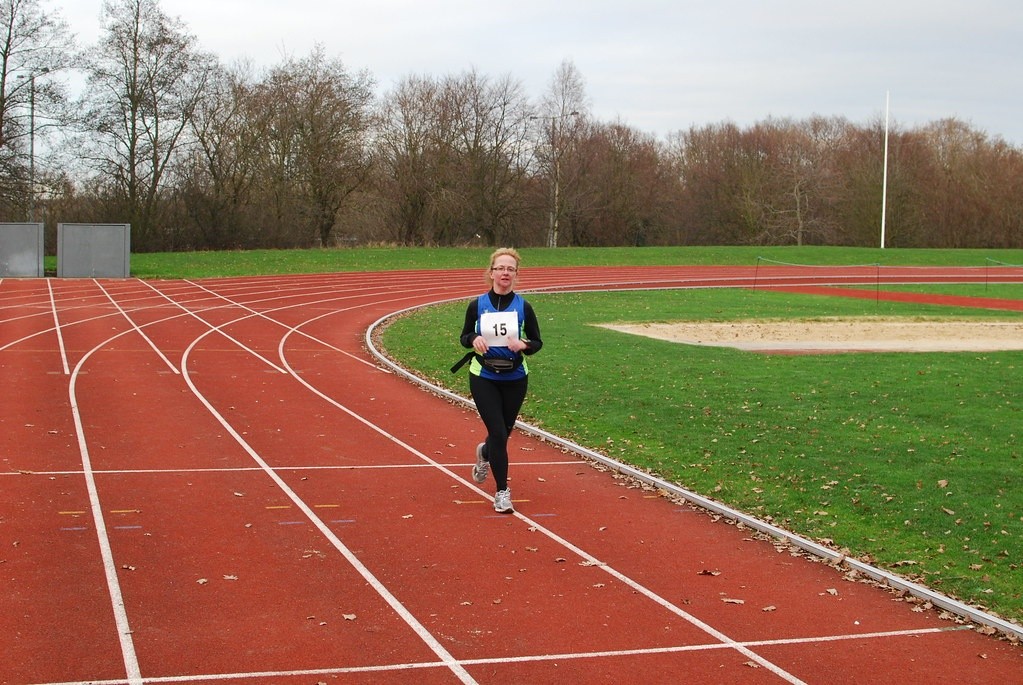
[{"xmin": 491, "ymin": 265, "xmax": 517, "ymax": 272}]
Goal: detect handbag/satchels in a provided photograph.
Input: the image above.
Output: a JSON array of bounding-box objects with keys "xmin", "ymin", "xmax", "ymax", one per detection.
[{"xmin": 476, "ymin": 354, "xmax": 520, "ymax": 373}]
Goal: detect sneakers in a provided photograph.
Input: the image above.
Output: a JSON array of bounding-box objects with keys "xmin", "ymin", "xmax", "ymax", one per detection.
[
  {"xmin": 472, "ymin": 443, "xmax": 490, "ymax": 483},
  {"xmin": 493, "ymin": 487, "xmax": 514, "ymax": 513}
]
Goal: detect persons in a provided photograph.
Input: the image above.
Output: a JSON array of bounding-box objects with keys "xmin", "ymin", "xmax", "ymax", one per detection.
[{"xmin": 460, "ymin": 247, "xmax": 542, "ymax": 514}]
